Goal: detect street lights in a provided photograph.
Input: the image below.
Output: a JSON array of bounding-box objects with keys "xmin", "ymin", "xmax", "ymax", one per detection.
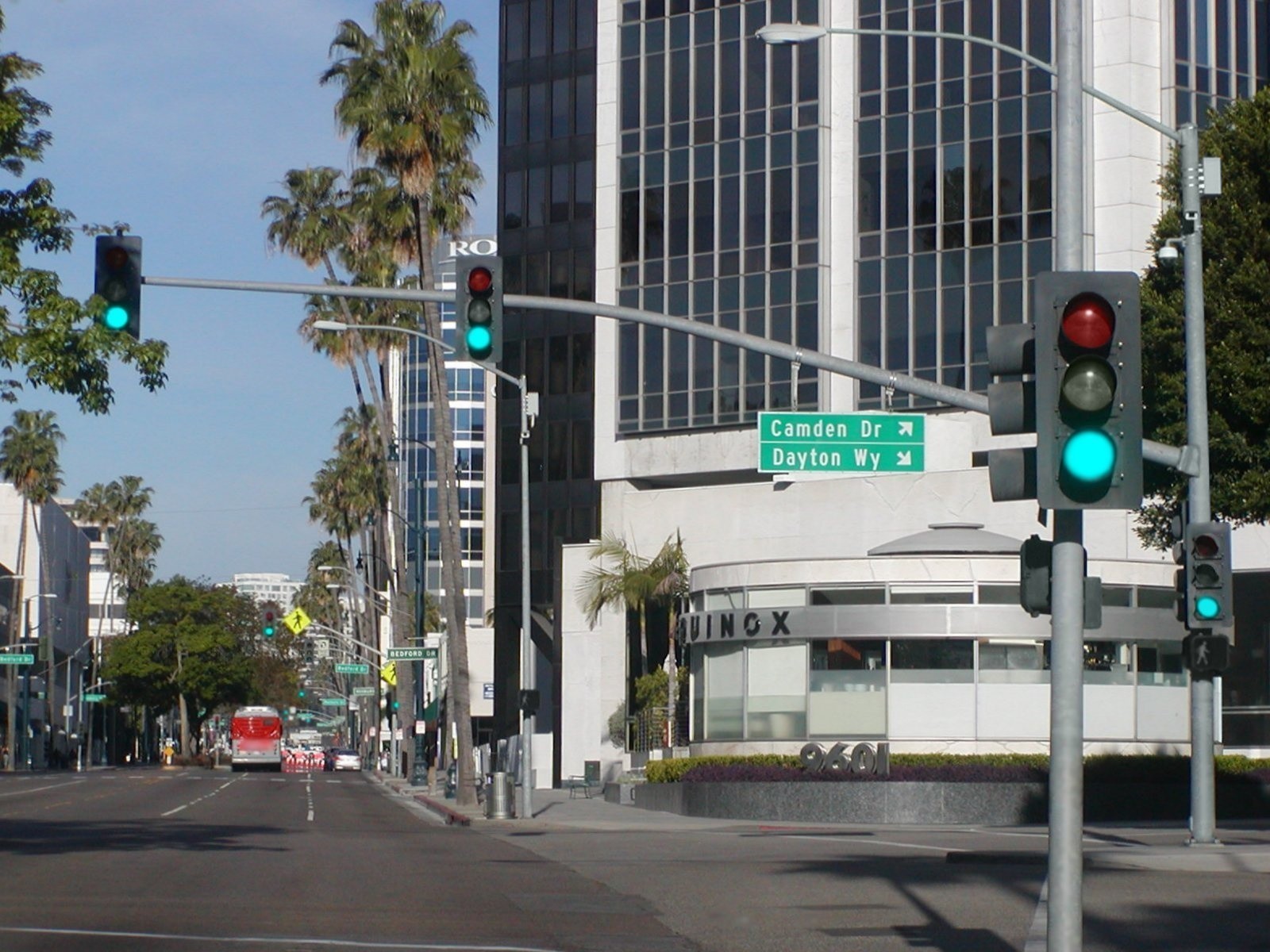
[
  {"xmin": 361, "ymin": 474, "xmax": 429, "ymax": 784},
  {"xmin": 756, "ymin": 22, "xmax": 1218, "ymax": 849},
  {"xmin": 67, "ymin": 623, "xmax": 140, "ymax": 767},
  {"xmin": 12, "ymin": 593, "xmax": 60, "ymax": 770},
  {"xmin": 386, "ymin": 428, "xmax": 463, "ymax": 512},
  {"xmin": 310, "ymin": 319, "xmax": 540, "ymax": 824}
]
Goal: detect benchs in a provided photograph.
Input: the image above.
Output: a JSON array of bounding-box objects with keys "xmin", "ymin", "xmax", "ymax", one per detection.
[{"xmin": 569, "ymin": 761, "xmax": 603, "ymax": 800}]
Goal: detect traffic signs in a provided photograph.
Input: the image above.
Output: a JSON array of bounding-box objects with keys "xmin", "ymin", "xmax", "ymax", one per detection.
[
  {"xmin": 757, "ymin": 412, "xmax": 928, "ymax": 439},
  {"xmin": 756, "ymin": 439, "xmax": 925, "ymax": 474}
]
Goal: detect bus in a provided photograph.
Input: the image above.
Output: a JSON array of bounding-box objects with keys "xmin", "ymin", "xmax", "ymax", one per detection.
[{"xmin": 230, "ymin": 706, "xmax": 283, "ymax": 769}]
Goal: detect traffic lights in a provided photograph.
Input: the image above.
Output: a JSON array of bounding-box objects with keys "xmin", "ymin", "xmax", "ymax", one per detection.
[
  {"xmin": 985, "ymin": 321, "xmax": 1040, "ymax": 503},
  {"xmin": 1169, "ymin": 496, "xmax": 1193, "ymax": 631},
  {"xmin": 1036, "ymin": 269, "xmax": 1143, "ymax": 512},
  {"xmin": 1016, "ymin": 538, "xmax": 1089, "ymax": 617},
  {"xmin": 518, "ymin": 690, "xmax": 543, "ymax": 711},
  {"xmin": 1184, "ymin": 523, "xmax": 1238, "ymax": 631},
  {"xmin": 92, "ymin": 233, "xmax": 145, "ymax": 339},
  {"xmin": 454, "ymin": 256, "xmax": 504, "ymax": 364},
  {"xmin": 262, "ymin": 604, "xmax": 279, "ymax": 642}
]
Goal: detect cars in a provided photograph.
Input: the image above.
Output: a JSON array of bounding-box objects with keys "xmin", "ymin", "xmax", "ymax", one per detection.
[{"xmin": 322, "ymin": 745, "xmax": 365, "ymax": 773}]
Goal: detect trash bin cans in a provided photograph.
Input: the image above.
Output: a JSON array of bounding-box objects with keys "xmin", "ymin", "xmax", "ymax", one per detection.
[
  {"xmin": 445, "ymin": 763, "xmax": 456, "ymax": 799},
  {"xmin": 485, "ymin": 772, "xmax": 516, "ymax": 818}
]
